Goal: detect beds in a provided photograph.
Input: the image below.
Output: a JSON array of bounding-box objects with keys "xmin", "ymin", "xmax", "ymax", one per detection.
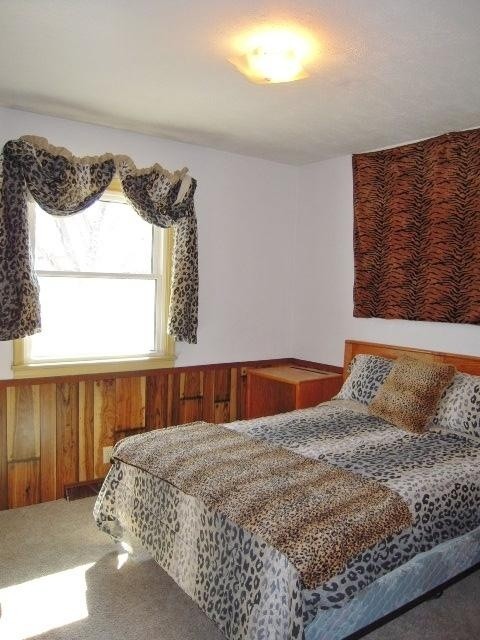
[{"xmin": 104, "ymin": 338, "xmax": 480, "ymax": 638}]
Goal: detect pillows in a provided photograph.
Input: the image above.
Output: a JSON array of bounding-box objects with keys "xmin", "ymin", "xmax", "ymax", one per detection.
[{"xmin": 330, "ymin": 353, "xmax": 480, "ymax": 449}]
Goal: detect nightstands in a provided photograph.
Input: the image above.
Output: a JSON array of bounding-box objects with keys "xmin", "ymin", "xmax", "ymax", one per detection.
[{"xmin": 243, "ymin": 364, "xmax": 344, "ymax": 420}]
